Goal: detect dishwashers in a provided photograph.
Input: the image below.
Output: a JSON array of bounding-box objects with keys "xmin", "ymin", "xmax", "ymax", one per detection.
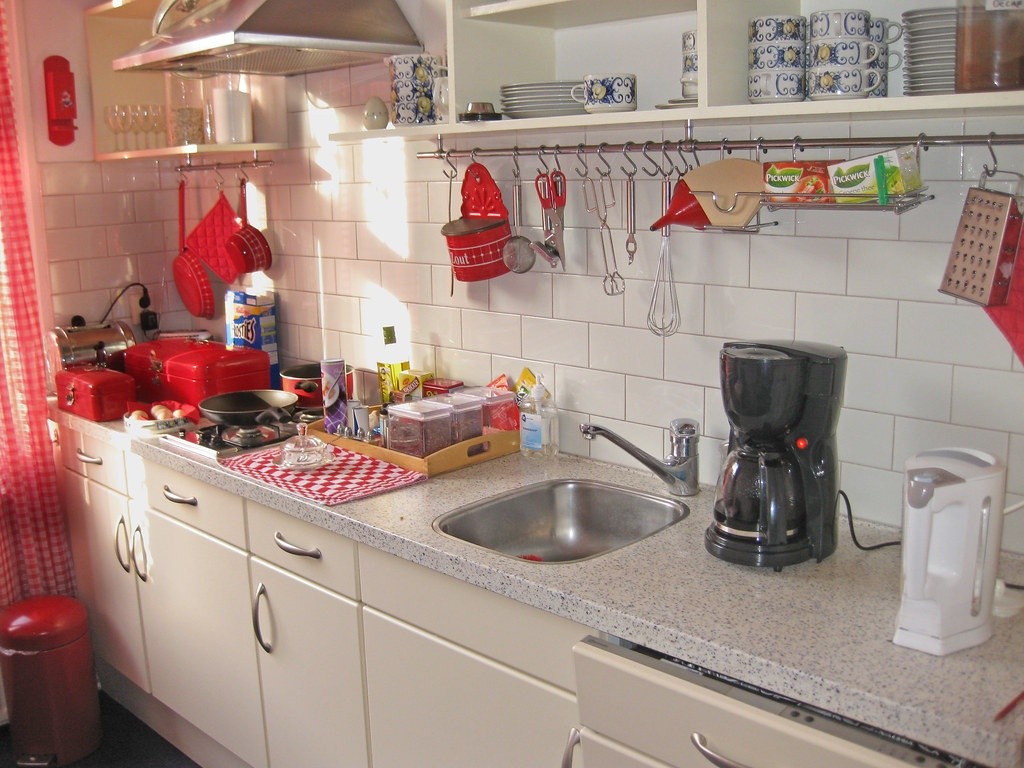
[{"xmin": 572, "ymin": 634, "xmax": 973, "ymax": 768}]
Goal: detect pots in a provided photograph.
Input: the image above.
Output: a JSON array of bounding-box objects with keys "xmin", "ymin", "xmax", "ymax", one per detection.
[
  {"xmin": 280, "ymin": 356, "xmax": 354, "ymax": 408},
  {"xmin": 225, "ymin": 178, "xmax": 273, "ymax": 275},
  {"xmin": 197, "ymin": 388, "xmax": 300, "ymax": 427},
  {"xmin": 172, "ymin": 179, "xmax": 215, "ymax": 320}
]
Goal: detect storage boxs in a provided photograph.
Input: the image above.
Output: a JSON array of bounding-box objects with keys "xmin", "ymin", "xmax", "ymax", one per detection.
[
  {"xmin": 396, "ymin": 370, "xmax": 434, "ymax": 400},
  {"xmin": 826, "ymin": 142, "xmax": 924, "ymax": 204},
  {"xmin": 429, "ymin": 394, "xmax": 486, "ymax": 444},
  {"xmin": 762, "ymin": 159, "xmax": 844, "ymax": 204},
  {"xmin": 423, "ymin": 377, "xmax": 464, "ymax": 398},
  {"xmin": 306, "ymin": 404, "xmax": 520, "ymax": 478},
  {"xmin": 385, "ymin": 396, "xmax": 453, "ymax": 456},
  {"xmin": 223, "ymin": 286, "xmax": 280, "ymax": 390},
  {"xmin": 455, "ymin": 384, "xmax": 527, "ymax": 431},
  {"xmin": 52, "ymin": 360, "xmax": 134, "ymax": 420},
  {"xmin": 123, "ymin": 337, "xmax": 273, "ymax": 418}
]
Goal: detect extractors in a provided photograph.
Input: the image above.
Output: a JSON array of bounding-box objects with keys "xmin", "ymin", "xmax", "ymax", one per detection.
[{"xmin": 112, "ymin": 0, "xmax": 424, "ymax": 78}]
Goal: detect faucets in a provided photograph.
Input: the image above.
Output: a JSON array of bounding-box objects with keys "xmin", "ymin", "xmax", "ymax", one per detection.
[{"xmin": 579, "ymin": 418, "xmax": 700, "ymax": 496}]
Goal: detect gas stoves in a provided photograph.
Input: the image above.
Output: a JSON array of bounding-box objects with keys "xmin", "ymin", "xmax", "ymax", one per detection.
[{"xmin": 160, "ymin": 395, "xmax": 323, "ymax": 458}]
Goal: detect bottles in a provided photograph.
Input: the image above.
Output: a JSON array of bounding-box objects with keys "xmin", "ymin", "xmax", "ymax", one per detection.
[
  {"xmin": 320, "ymin": 324, "xmax": 516, "ymax": 456},
  {"xmin": 167, "ymin": 64, "xmax": 255, "ymax": 143}
]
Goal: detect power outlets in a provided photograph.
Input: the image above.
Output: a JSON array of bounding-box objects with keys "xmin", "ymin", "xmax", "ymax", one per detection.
[{"xmin": 129, "ymin": 292, "xmax": 154, "ymax": 326}]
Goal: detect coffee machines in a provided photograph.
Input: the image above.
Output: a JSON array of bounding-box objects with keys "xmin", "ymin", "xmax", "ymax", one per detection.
[{"xmin": 704, "ymin": 338, "xmax": 849, "ymax": 566}]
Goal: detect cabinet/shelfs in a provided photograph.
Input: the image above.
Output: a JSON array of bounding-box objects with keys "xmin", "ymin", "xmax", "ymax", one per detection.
[
  {"xmin": 82, "ymin": 0, "xmax": 291, "ymax": 165},
  {"xmin": 246, "ymin": 492, "xmax": 371, "ymax": 768},
  {"xmin": 330, "ymin": 0, "xmax": 1024, "ymax": 144},
  {"xmin": 368, "ymin": 536, "xmax": 598, "ymax": 768},
  {"xmin": 55, "ymin": 424, "xmax": 268, "ymax": 768},
  {"xmin": 571, "ymin": 620, "xmax": 941, "ymax": 768}
]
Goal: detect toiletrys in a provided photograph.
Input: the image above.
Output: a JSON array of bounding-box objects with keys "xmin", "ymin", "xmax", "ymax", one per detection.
[{"xmin": 519, "ymin": 371, "xmax": 559, "ymax": 459}]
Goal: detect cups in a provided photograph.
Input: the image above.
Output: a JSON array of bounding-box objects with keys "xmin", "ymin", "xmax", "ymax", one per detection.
[
  {"xmin": 570, "ymin": 72, "xmax": 636, "ymax": 113},
  {"xmin": 382, "ymin": 52, "xmax": 449, "ymax": 127},
  {"xmin": 680, "ymin": 30, "xmax": 698, "ymax": 98},
  {"xmin": 747, "ymin": 9, "xmax": 902, "ymax": 104}
]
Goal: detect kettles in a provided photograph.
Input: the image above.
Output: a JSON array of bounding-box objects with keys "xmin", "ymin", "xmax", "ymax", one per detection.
[{"xmin": 891, "ymin": 446, "xmax": 1006, "ymax": 657}]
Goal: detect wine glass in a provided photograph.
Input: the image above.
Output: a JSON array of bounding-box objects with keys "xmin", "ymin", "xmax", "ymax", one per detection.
[{"xmin": 105, "ymin": 103, "xmax": 168, "ymax": 152}]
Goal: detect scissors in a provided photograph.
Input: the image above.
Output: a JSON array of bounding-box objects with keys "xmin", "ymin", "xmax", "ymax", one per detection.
[{"xmin": 535, "ymin": 172, "xmax": 566, "ymax": 271}]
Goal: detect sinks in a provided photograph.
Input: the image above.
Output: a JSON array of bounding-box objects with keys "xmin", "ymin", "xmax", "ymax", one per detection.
[{"xmin": 431, "ymin": 478, "xmax": 690, "ymax": 565}]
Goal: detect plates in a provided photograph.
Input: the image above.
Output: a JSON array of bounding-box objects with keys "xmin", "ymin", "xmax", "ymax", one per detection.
[
  {"xmin": 497, "ymin": 81, "xmax": 584, "ymax": 119},
  {"xmin": 902, "ymin": 6, "xmax": 1016, "ymax": 96},
  {"xmin": 654, "ymin": 96, "xmax": 698, "ymax": 108}
]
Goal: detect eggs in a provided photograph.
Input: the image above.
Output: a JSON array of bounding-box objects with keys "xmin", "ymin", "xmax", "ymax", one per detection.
[
  {"xmin": 363, "ymin": 97, "xmax": 389, "ymax": 130},
  {"xmin": 129, "ymin": 405, "xmax": 184, "ymax": 420}
]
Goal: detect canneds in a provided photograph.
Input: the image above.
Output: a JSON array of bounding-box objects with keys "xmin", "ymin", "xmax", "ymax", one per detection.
[{"xmin": 320, "ymin": 358, "xmax": 348, "ymax": 434}]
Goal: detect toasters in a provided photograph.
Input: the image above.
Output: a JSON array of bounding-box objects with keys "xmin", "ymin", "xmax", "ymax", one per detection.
[{"xmin": 42, "ymin": 320, "xmax": 136, "ymax": 395}]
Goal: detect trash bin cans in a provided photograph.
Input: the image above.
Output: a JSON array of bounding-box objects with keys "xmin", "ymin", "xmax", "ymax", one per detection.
[{"xmin": 0, "ymin": 595, "xmax": 103, "ymax": 768}]
